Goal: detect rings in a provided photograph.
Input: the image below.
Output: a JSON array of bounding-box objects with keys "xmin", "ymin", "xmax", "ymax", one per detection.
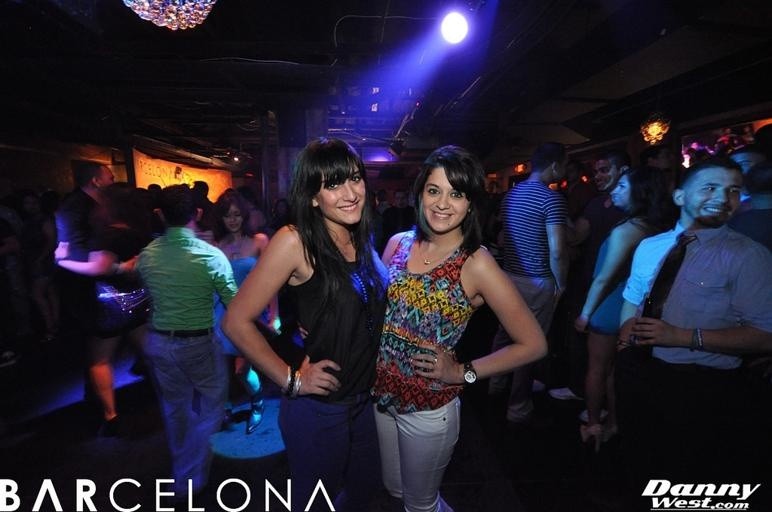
[{"xmin": 431, "ymin": 355, "xmax": 439, "ymax": 365}]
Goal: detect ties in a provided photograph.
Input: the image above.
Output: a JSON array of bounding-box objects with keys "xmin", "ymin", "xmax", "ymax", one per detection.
[{"xmin": 636, "ymin": 232, "xmax": 698, "ymax": 355}]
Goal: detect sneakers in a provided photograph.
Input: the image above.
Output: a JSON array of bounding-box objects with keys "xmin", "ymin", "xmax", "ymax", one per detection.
[
  {"xmin": 96, "ymin": 416, "xmax": 123, "ymax": 436},
  {"xmin": 548, "ymin": 386, "xmax": 585, "ymax": 403},
  {"xmin": 506, "ymin": 415, "xmax": 554, "ymax": 433},
  {"xmin": 532, "ymin": 380, "xmax": 546, "ymax": 394}
]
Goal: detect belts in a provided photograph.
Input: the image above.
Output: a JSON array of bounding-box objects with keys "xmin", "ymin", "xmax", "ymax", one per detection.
[{"xmin": 151, "ymin": 326, "xmax": 214, "ymax": 338}]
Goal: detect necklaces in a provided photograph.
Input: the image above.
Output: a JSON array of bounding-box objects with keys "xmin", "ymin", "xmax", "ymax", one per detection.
[{"xmin": 421, "ymin": 236, "xmax": 459, "ymax": 266}]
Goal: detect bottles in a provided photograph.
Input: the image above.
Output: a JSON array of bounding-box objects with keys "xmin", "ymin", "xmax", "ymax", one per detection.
[{"xmin": 631, "ymin": 300, "xmax": 654, "ymax": 364}]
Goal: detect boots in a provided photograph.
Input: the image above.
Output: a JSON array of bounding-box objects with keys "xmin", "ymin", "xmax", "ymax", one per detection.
[{"xmin": 246, "ymin": 393, "xmax": 265, "ymax": 434}]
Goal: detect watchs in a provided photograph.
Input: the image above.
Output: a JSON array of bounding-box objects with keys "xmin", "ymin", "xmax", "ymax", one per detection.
[{"xmin": 459, "ymin": 358, "xmax": 478, "ymax": 386}]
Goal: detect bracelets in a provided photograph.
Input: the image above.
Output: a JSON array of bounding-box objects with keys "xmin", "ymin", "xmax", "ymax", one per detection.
[{"xmin": 281, "ymin": 363, "xmax": 303, "ymax": 397}]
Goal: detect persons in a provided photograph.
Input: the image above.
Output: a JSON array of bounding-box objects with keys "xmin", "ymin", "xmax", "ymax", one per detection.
[
  {"xmin": 374, "ymin": 145, "xmax": 552, "ymax": 511},
  {"xmin": 373, "ymin": 186, "xmax": 414, "ymax": 248},
  {"xmin": 0, "ymin": 157, "xmax": 286, "ymax": 512},
  {"xmin": 224, "ymin": 135, "xmax": 390, "ymax": 512},
  {"xmin": 482, "ymin": 120, "xmax": 770, "ymax": 512}
]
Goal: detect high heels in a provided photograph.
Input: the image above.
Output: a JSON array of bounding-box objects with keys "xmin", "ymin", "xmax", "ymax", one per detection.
[
  {"xmin": 579, "ymin": 422, "xmax": 602, "ymax": 452},
  {"xmin": 603, "ymin": 426, "xmax": 618, "ymax": 444}
]
